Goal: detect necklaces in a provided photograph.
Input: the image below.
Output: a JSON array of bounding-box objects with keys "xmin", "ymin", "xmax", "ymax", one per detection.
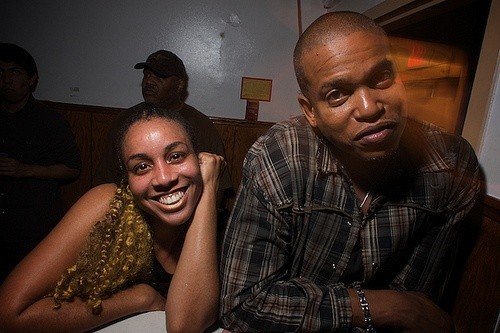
[{"xmin": 360, "ymin": 184, "xmax": 374, "ymax": 209}]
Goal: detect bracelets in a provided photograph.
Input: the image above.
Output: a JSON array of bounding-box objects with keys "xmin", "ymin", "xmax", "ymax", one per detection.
[{"xmin": 353, "ymin": 281, "xmax": 375, "ymax": 333}]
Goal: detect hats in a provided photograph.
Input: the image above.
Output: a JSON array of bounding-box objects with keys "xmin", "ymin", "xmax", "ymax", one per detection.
[{"xmin": 134, "ymin": 49, "xmax": 186, "ymax": 77}]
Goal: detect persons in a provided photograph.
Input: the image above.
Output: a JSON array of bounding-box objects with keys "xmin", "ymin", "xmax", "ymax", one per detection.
[
  {"xmin": 105, "ymin": 50, "xmax": 234, "ymax": 190},
  {"xmin": 218, "ymin": 12, "xmax": 488, "ymax": 333},
  {"xmin": 0, "ymin": 42, "xmax": 87, "ymax": 263},
  {"xmin": 0, "ymin": 105, "xmax": 228, "ymax": 333}
]
